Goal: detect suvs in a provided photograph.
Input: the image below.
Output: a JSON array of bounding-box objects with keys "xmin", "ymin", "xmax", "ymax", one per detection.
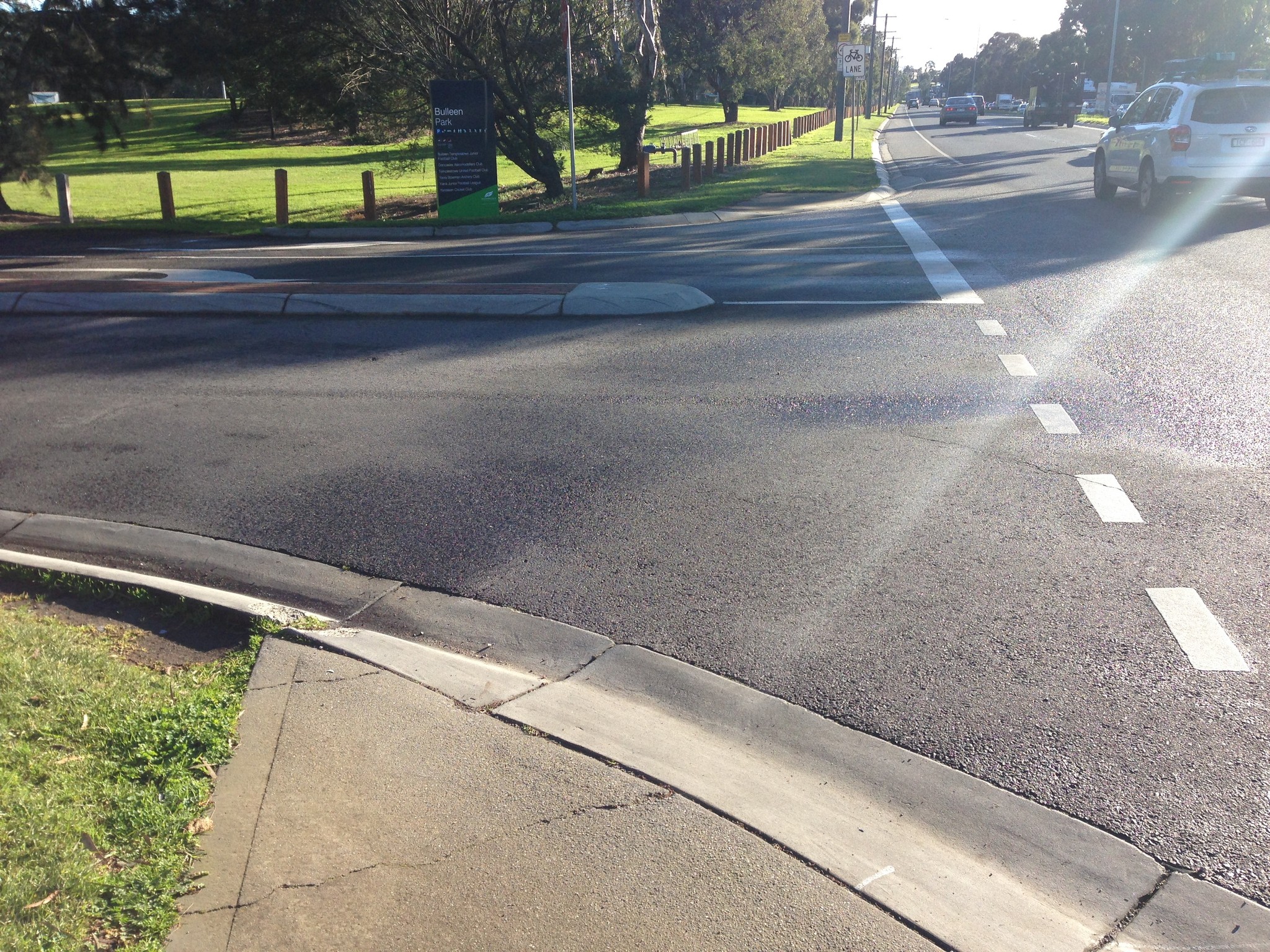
[
  {"xmin": 1093, "ymin": 75, "xmax": 1270, "ymax": 212},
  {"xmin": 907, "ymin": 99, "xmax": 919, "ymax": 110}
]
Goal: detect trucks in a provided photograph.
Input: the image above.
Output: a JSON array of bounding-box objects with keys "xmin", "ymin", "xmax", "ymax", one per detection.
[
  {"xmin": 1082, "ymin": 80, "xmax": 1140, "ymax": 118},
  {"xmin": 1023, "ymin": 70, "xmax": 1086, "ymax": 128}
]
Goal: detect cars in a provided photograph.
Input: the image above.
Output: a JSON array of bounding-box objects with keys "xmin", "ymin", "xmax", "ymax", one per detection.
[
  {"xmin": 985, "ymin": 94, "xmax": 1027, "ymax": 113},
  {"xmin": 929, "ymin": 99, "xmax": 940, "ymax": 107},
  {"xmin": 939, "ymin": 96, "xmax": 985, "ymax": 125}
]
